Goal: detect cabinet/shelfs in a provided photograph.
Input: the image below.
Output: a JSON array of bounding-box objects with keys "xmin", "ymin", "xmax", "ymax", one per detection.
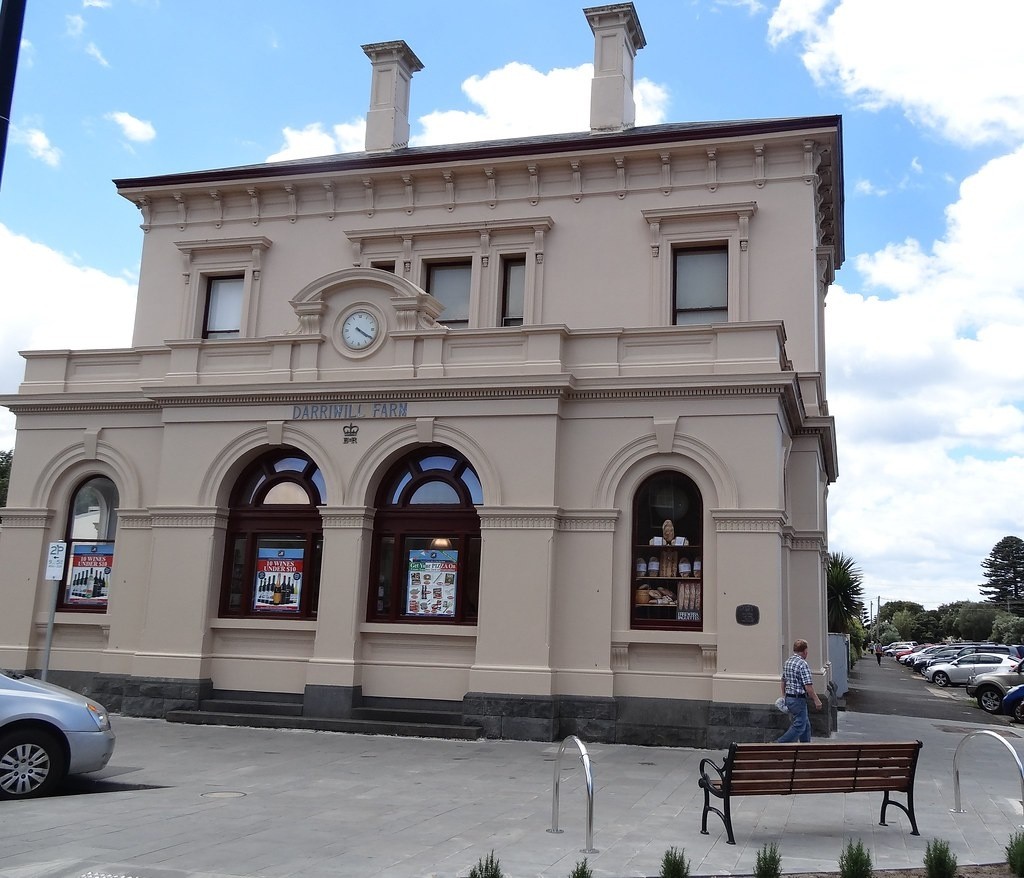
[{"xmin": 631, "ymin": 545, "xmax": 703, "ymax": 627}]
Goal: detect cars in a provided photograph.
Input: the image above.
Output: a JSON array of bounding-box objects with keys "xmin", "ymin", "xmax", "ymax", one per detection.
[
  {"xmin": 882, "ymin": 641, "xmax": 1024, "ymax": 723},
  {"xmin": 0, "ymin": 667, "xmax": 116, "ymax": 799}
]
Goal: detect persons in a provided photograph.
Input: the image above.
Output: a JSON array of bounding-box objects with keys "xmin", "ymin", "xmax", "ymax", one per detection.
[
  {"xmin": 868, "ymin": 641, "xmax": 875, "ymax": 654},
  {"xmin": 874, "ymin": 639, "xmax": 883, "ymax": 665},
  {"xmin": 776, "ymin": 638, "xmax": 823, "ymax": 743}
]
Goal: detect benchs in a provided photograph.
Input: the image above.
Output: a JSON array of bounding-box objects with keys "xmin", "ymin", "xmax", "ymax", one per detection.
[{"xmin": 699, "ymin": 740, "xmax": 923, "ymax": 845}]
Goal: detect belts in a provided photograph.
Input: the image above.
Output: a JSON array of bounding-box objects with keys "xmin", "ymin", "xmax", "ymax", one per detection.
[{"xmin": 786, "ymin": 693, "xmax": 807, "ymax": 698}]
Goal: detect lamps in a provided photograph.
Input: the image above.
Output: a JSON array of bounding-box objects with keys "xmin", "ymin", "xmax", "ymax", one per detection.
[{"xmin": 430, "ymin": 539, "xmax": 452, "ymax": 549}]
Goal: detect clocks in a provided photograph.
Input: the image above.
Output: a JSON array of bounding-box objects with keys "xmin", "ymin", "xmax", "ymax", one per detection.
[{"xmin": 340, "ymin": 310, "xmax": 379, "ymax": 350}]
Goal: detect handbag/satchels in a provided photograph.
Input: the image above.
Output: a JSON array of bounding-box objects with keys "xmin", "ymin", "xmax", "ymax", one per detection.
[{"xmin": 882, "ymin": 652, "xmax": 884, "ymax": 656}]
